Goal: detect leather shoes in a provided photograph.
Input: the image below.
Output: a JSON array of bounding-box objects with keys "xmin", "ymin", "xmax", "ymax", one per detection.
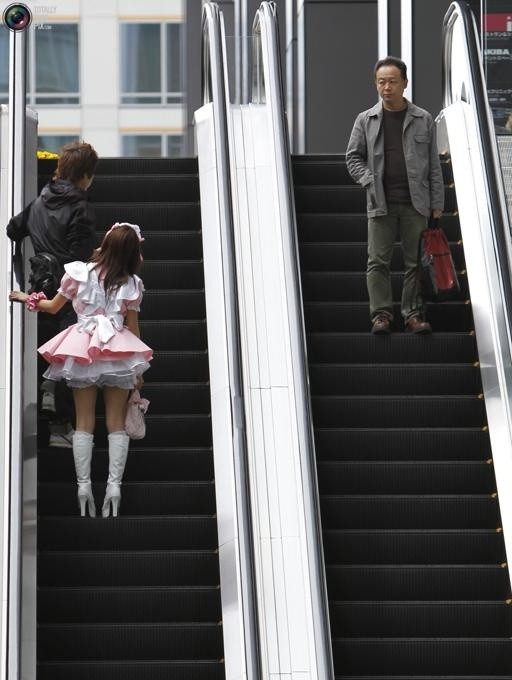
[
  {"xmin": 405, "ymin": 316, "xmax": 433, "ymax": 333},
  {"xmin": 371, "ymin": 316, "xmax": 392, "ymax": 334}
]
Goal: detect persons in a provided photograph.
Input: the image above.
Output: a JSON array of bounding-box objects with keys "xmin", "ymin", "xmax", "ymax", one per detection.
[
  {"xmin": 8, "ymin": 222, "xmax": 154, "ymax": 518},
  {"xmin": 344, "ymin": 57, "xmax": 445, "ymax": 334},
  {"xmin": 5, "ymin": 141, "xmax": 98, "ymax": 449}
]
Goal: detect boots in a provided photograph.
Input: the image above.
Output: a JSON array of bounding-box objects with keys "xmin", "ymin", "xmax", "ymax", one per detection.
[
  {"xmin": 101, "ymin": 431, "xmax": 130, "ymax": 519},
  {"xmin": 73, "ymin": 431, "xmax": 96, "ymax": 518}
]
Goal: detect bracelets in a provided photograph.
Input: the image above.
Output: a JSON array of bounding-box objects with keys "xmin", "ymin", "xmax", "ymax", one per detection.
[{"xmin": 26, "ymin": 292, "xmax": 46, "ymax": 313}]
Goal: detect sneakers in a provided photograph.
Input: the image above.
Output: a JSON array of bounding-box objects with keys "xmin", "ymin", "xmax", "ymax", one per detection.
[
  {"xmin": 41, "ymin": 392, "xmax": 56, "ymax": 412},
  {"xmin": 48, "ymin": 428, "xmax": 95, "ymax": 448}
]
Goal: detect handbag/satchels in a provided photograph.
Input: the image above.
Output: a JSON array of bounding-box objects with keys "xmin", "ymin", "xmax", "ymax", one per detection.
[
  {"xmin": 28, "ymin": 252, "xmax": 60, "ymax": 300},
  {"xmin": 124, "ymin": 389, "xmax": 151, "ymax": 440},
  {"xmin": 419, "ymin": 227, "xmax": 462, "ymax": 302}
]
